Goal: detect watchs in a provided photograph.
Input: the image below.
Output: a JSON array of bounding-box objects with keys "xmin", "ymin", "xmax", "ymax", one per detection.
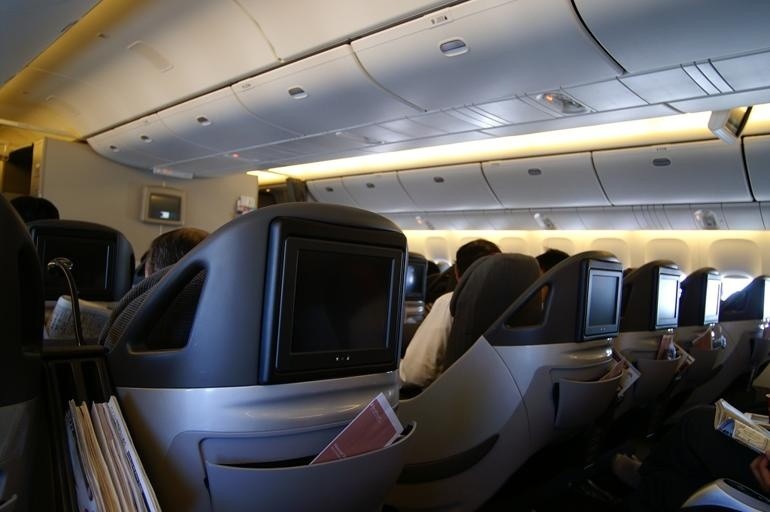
[{"xmin": 535, "ymin": 249, "xmax": 570, "ymax": 310}]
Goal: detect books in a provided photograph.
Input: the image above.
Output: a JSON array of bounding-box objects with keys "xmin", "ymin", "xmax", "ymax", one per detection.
[
  {"xmin": 715, "ymin": 399, "xmax": 769, "ymax": 458},
  {"xmin": 67, "ymin": 396, "xmax": 164, "ymax": 512},
  {"xmin": 598, "ymin": 324, "xmax": 768, "ymax": 398}
]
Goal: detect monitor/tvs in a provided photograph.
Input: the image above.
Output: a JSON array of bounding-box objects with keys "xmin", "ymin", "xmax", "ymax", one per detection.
[
  {"xmin": 405, "ymin": 256, "xmax": 429, "ymax": 300},
  {"xmin": 276, "ymin": 236, "xmax": 404, "ymax": 371},
  {"xmin": 704, "ymin": 275, "xmax": 722, "ymax": 325},
  {"xmin": 656, "ymin": 273, "xmax": 680, "ymax": 327},
  {"xmin": 584, "ymin": 269, "xmax": 623, "ymax": 336},
  {"xmin": 140, "ymin": 184, "xmax": 187, "ymax": 226},
  {"xmin": 36, "ymin": 231, "xmax": 116, "ymax": 302}
]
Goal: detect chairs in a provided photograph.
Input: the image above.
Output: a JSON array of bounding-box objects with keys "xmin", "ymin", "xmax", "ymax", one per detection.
[
  {"xmin": 614, "ymin": 257, "xmax": 684, "ymax": 434},
  {"xmin": 709, "ymin": 275, "xmax": 769, "ymax": 409},
  {"xmin": 402, "ymin": 250, "xmax": 626, "ymax": 511},
  {"xmin": 404, "ymin": 250, "xmax": 450, "ymax": 336},
  {"xmin": 0, "ymin": 197, "xmax": 136, "ymax": 511},
  {"xmin": 679, "ymin": 266, "xmax": 721, "ymax": 406},
  {"xmin": 94, "ymin": 203, "xmax": 421, "ymax": 511}
]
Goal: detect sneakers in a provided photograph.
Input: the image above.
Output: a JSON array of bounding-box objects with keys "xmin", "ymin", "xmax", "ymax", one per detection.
[{"xmin": 614, "ymin": 454, "xmax": 642, "ymax": 488}]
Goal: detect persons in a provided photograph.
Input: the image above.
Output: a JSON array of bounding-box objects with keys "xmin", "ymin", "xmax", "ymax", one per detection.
[
  {"xmin": 143, "ymin": 227, "xmax": 211, "ymax": 278},
  {"xmin": 399, "ymin": 239, "xmax": 501, "ymax": 398},
  {"xmin": 612, "ymin": 403, "xmax": 769, "ymax": 512}
]
